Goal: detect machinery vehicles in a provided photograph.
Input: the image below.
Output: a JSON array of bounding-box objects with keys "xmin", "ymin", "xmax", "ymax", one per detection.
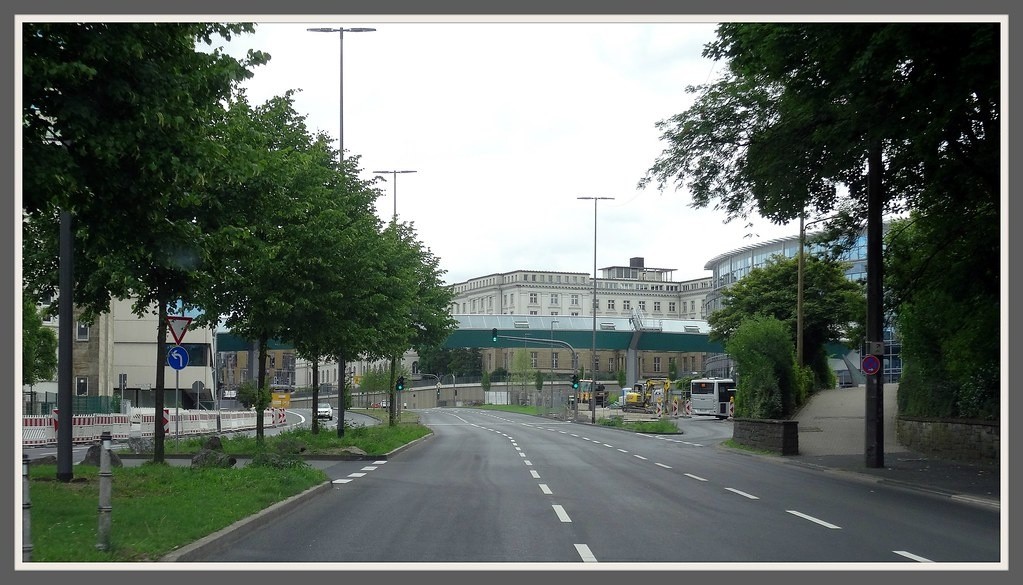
[
  {"xmin": 568, "ymin": 379, "xmax": 609, "ymax": 411},
  {"xmin": 618, "ymin": 377, "xmax": 670, "ymax": 414}
]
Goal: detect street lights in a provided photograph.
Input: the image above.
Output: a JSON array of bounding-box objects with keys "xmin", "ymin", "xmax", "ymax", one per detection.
[
  {"xmin": 373, "ymin": 170, "xmax": 418, "ymax": 426},
  {"xmin": 306, "ymin": 27, "xmax": 379, "ymax": 438},
  {"xmin": 797, "ymin": 212, "xmax": 844, "ymax": 369},
  {"xmin": 550, "ymin": 319, "xmax": 559, "ymax": 409},
  {"xmin": 575, "ymin": 196, "xmax": 615, "ymax": 425}
]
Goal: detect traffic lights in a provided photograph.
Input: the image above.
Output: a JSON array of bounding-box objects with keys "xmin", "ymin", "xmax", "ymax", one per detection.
[
  {"xmin": 573, "ymin": 373, "xmax": 579, "ymax": 390},
  {"xmin": 396, "ymin": 379, "xmax": 403, "ymax": 391},
  {"xmin": 491, "ymin": 328, "xmax": 497, "ymax": 342}
]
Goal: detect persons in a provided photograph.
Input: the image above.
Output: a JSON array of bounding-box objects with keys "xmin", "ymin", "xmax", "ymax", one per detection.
[{"xmin": 404, "ymin": 402, "xmax": 407, "ymax": 409}]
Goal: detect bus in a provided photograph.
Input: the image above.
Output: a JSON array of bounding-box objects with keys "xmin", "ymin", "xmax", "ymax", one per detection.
[{"xmin": 690, "ymin": 377, "xmax": 734, "ymax": 420}]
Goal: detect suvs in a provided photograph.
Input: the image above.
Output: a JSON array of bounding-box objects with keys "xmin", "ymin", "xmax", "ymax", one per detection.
[
  {"xmin": 380, "ymin": 400, "xmax": 390, "ymax": 408},
  {"xmin": 311, "ymin": 402, "xmax": 334, "ymax": 421}
]
